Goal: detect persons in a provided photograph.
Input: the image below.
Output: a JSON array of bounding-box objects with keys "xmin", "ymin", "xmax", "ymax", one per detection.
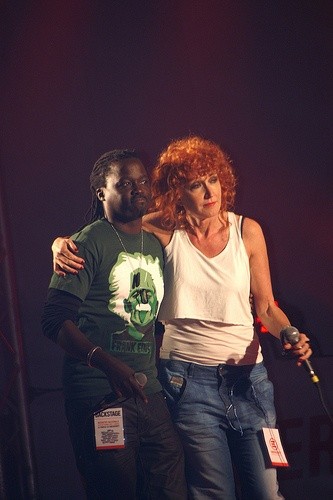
[
  {"xmin": 51, "ymin": 137, "xmax": 312, "ymax": 500},
  {"xmin": 40, "ymin": 151, "xmax": 188, "ymax": 500}
]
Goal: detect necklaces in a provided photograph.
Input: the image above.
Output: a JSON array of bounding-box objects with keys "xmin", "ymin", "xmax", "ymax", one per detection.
[{"xmin": 111, "ymin": 223, "xmax": 144, "ymax": 288}]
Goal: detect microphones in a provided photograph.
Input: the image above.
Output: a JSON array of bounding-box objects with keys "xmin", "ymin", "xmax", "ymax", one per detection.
[
  {"xmin": 280, "ymin": 325, "xmax": 320, "ymax": 384},
  {"xmin": 90, "ymin": 373, "xmax": 148, "ymax": 414}
]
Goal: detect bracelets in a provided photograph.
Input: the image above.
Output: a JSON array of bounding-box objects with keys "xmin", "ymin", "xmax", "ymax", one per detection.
[{"xmin": 86, "ymin": 346, "xmax": 99, "ymax": 369}]
